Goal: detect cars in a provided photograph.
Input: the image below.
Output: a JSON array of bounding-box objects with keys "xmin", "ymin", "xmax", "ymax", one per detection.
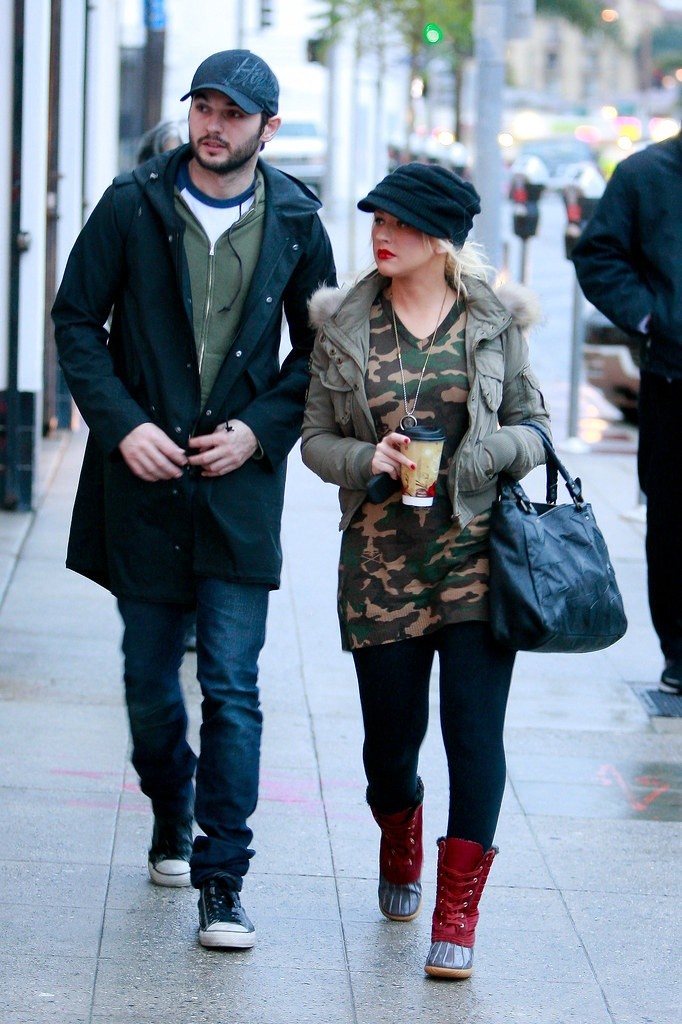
[
  {"xmin": 258, "ymin": 105, "xmax": 327, "ymax": 194},
  {"xmin": 586, "ymin": 312, "xmax": 640, "ymax": 421}
]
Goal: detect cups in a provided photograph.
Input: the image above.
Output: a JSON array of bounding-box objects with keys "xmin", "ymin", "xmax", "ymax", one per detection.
[{"xmin": 398, "ymin": 425, "xmax": 447, "ymax": 507}]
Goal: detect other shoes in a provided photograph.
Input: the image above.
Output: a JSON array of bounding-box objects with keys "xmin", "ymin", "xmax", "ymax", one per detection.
[{"xmin": 658, "ymin": 668, "xmax": 682, "ymax": 693}]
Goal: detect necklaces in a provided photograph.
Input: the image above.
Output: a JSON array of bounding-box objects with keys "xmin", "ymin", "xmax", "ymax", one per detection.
[{"xmin": 388, "ymin": 281, "xmax": 449, "ymax": 431}]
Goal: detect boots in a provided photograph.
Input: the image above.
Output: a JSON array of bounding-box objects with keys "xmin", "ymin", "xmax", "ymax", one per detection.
[
  {"xmin": 424, "ymin": 836, "xmax": 500, "ymax": 978},
  {"xmin": 365, "ymin": 776, "xmax": 425, "ymax": 922}
]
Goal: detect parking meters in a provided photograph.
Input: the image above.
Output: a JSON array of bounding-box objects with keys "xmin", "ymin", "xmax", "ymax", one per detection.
[
  {"xmin": 556, "ymin": 167, "xmax": 606, "ymax": 441},
  {"xmin": 506, "ymin": 155, "xmax": 549, "ymax": 288}
]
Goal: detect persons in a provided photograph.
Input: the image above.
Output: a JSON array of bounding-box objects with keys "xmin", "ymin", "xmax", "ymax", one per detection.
[
  {"xmin": 302, "ymin": 162, "xmax": 555, "ymax": 980},
  {"xmin": 135, "ymin": 108, "xmax": 284, "ymax": 650},
  {"xmin": 573, "ymin": 129, "xmax": 682, "ymax": 692},
  {"xmin": 50, "ymin": 47, "xmax": 338, "ymax": 949}
]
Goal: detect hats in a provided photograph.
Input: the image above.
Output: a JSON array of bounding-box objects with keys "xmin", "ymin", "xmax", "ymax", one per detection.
[
  {"xmin": 357, "ymin": 161, "xmax": 481, "ymax": 249},
  {"xmin": 180, "ymin": 49, "xmax": 280, "ymax": 116}
]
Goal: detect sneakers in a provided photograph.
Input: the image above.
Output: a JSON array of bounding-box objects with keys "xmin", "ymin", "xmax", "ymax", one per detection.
[
  {"xmin": 147, "ymin": 815, "xmax": 194, "ymax": 887},
  {"xmin": 197, "ymin": 886, "xmax": 257, "ymax": 948}
]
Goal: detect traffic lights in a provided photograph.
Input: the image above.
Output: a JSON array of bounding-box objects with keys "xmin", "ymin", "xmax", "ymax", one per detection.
[{"xmin": 424, "ymin": 21, "xmax": 444, "ymax": 46}]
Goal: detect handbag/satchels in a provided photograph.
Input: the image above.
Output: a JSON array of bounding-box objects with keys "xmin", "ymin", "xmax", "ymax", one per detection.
[{"xmin": 474, "ymin": 419, "xmax": 628, "ymax": 654}]
